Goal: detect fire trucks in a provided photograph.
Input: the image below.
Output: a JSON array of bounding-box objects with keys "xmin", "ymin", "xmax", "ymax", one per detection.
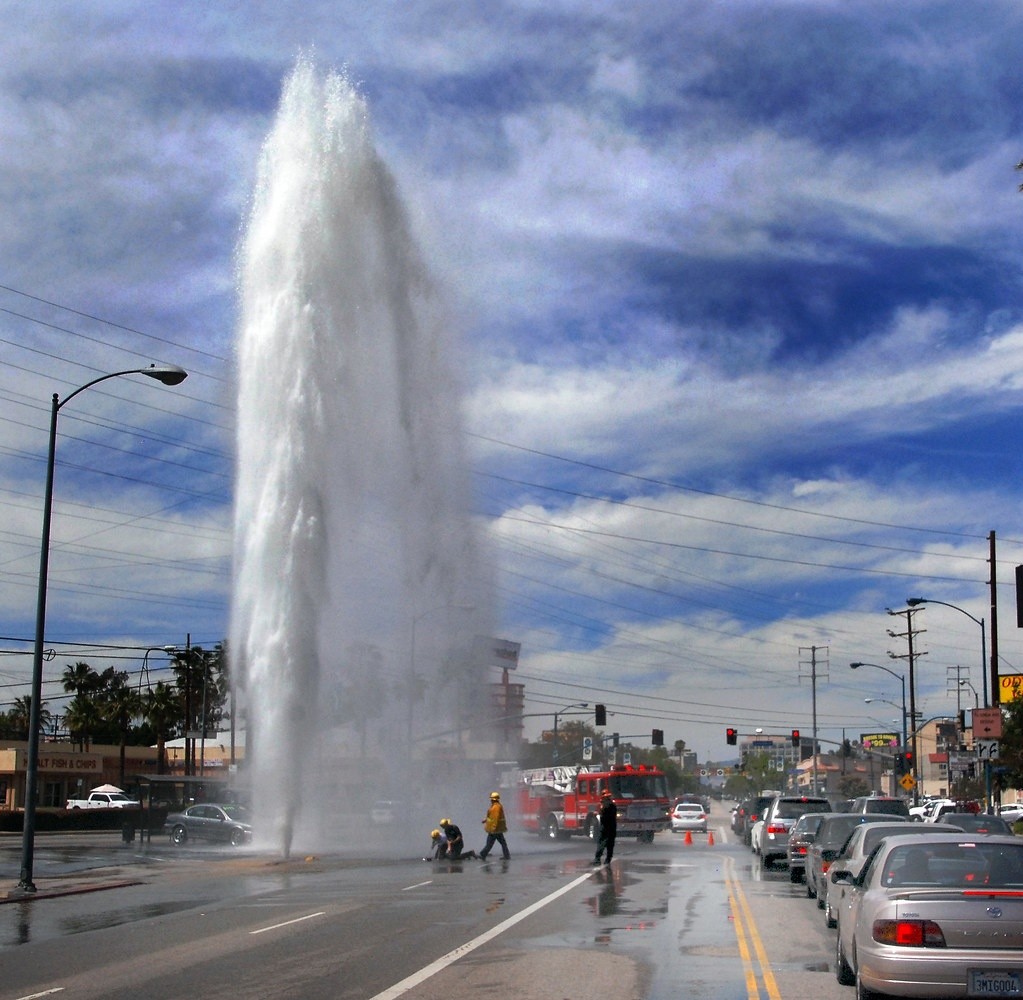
[{"xmin": 518, "ymin": 764, "xmax": 673, "ymax": 845}]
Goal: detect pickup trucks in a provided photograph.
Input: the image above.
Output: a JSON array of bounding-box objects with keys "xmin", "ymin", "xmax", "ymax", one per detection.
[{"xmin": 66, "ymin": 792, "xmax": 140, "ymax": 809}]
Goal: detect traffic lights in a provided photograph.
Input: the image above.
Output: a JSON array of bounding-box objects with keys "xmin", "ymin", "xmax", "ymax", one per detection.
[
  {"xmin": 726, "ymin": 728, "xmax": 734, "ymax": 744},
  {"xmin": 792, "ymin": 729, "xmax": 800, "ymax": 747},
  {"xmin": 894, "ymin": 752, "xmax": 913, "ymax": 777}
]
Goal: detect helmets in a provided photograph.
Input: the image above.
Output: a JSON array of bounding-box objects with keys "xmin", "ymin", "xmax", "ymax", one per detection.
[
  {"xmin": 431, "ymin": 830, "xmax": 440, "ymax": 838},
  {"xmin": 440, "ymin": 819, "xmax": 447, "ymax": 827},
  {"xmin": 489, "ymin": 792, "xmax": 500, "ymax": 800},
  {"xmin": 600, "ymin": 789, "xmax": 612, "ymax": 798}
]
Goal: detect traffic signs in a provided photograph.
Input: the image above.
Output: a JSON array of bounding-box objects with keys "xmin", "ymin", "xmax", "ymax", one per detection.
[{"xmin": 972, "ymin": 708, "xmax": 1003, "ymax": 739}]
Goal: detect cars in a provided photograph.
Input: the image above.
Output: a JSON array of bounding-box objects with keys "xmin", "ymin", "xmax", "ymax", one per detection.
[
  {"xmin": 830, "ymin": 832, "xmax": 1023, "ymax": 1000},
  {"xmin": 934, "ymin": 811, "xmax": 1014, "ymax": 836},
  {"xmin": 668, "ymin": 794, "xmax": 711, "ymax": 817},
  {"xmin": 907, "ymin": 793, "xmax": 958, "ymax": 823},
  {"xmin": 821, "ymin": 822, "xmax": 991, "ymax": 927},
  {"xmin": 670, "ymin": 803, "xmax": 707, "ymax": 834},
  {"xmin": 729, "ymin": 796, "xmax": 774, "ymax": 856},
  {"xmin": 370, "ymin": 799, "xmax": 405, "ymax": 825},
  {"xmin": 981, "ymin": 804, "xmax": 1023, "ymax": 825},
  {"xmin": 802, "ymin": 813, "xmax": 906, "ymax": 910},
  {"xmin": 163, "ymin": 802, "xmax": 252, "ymax": 847},
  {"xmin": 784, "ymin": 814, "xmax": 828, "ymax": 883}
]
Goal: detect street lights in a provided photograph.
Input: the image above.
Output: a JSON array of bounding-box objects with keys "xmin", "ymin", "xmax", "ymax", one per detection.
[
  {"xmin": 904, "ymin": 597, "xmax": 993, "ymax": 816},
  {"xmin": 163, "ymin": 644, "xmax": 209, "ymax": 776},
  {"xmin": 848, "ymin": 662, "xmax": 909, "ymax": 796},
  {"xmin": 958, "ymin": 678, "xmax": 979, "ymax": 709},
  {"xmin": 15, "ymin": 361, "xmax": 190, "ymax": 893},
  {"xmin": 553, "ymin": 703, "xmax": 589, "ymax": 766},
  {"xmin": 405, "ymin": 599, "xmax": 478, "ymax": 804}
]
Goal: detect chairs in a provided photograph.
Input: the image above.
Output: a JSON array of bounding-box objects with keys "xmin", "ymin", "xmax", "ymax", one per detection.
[
  {"xmin": 891, "ymin": 849, "xmax": 937, "ymax": 886},
  {"xmin": 989, "ymin": 857, "xmax": 1015, "ymax": 886}
]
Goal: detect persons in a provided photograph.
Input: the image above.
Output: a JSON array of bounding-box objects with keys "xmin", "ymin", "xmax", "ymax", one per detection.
[
  {"xmin": 429, "ymin": 829, "xmax": 448, "ymax": 861},
  {"xmin": 441, "ymin": 817, "xmax": 479, "ymax": 860},
  {"xmin": 588, "ymin": 789, "xmax": 618, "ymax": 866},
  {"xmin": 477, "ymin": 792, "xmax": 512, "ymax": 860}
]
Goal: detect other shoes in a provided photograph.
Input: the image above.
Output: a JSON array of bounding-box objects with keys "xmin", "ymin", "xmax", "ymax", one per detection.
[
  {"xmin": 591, "ymin": 859, "xmax": 602, "ymax": 865},
  {"xmin": 470, "ymin": 850, "xmax": 477, "ymax": 859},
  {"xmin": 499, "ymin": 857, "xmax": 512, "ymax": 861},
  {"xmin": 478, "ymin": 855, "xmax": 485, "ymax": 859}
]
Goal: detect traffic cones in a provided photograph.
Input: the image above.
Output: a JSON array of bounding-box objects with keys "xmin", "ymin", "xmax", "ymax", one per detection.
[
  {"xmin": 708, "ymin": 832, "xmax": 714, "ymax": 846},
  {"xmin": 684, "ymin": 830, "xmax": 692, "ymax": 845}
]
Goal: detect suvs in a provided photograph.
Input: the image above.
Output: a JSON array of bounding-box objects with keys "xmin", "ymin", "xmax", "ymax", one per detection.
[
  {"xmin": 850, "ymin": 796, "xmax": 911, "ymax": 819},
  {"xmin": 756, "ymin": 796, "xmax": 833, "ymax": 871}
]
